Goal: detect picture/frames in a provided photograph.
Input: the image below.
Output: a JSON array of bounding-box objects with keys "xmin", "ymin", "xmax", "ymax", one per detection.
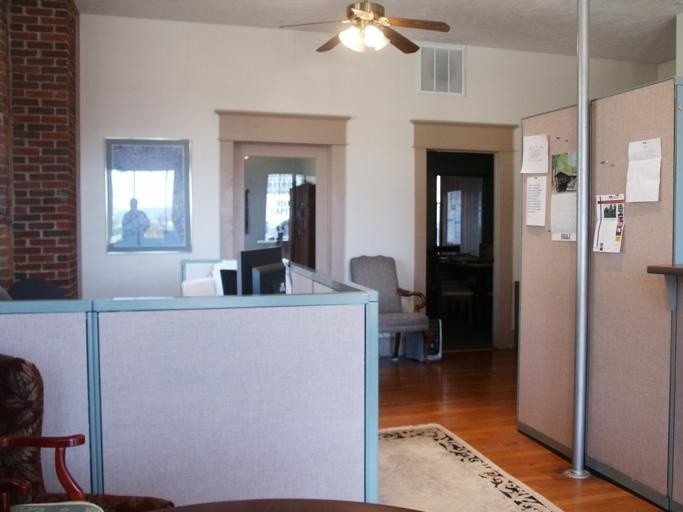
[{"xmin": 104, "ymin": 137, "xmax": 192, "ymax": 252}]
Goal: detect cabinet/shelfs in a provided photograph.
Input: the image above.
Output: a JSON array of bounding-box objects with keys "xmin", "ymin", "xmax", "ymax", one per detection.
[{"xmin": 290, "ymin": 183, "xmax": 315, "ymax": 270}]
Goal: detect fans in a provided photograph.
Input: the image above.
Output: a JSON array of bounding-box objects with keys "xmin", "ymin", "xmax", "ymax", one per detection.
[{"xmin": 280, "ymin": 0, "xmax": 450, "ymax": 53}]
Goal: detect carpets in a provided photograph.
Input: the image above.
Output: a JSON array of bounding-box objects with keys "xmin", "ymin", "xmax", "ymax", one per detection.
[{"xmin": 378, "ymin": 423, "xmax": 566, "ymax": 512}]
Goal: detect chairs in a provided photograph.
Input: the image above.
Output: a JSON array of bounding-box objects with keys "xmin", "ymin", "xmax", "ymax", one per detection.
[
  {"xmin": 351, "ymin": 255, "xmax": 432, "ymax": 366},
  {"xmin": 0, "ymin": 354, "xmax": 174, "ymax": 512},
  {"xmin": 7, "ymin": 280, "xmax": 68, "ymax": 300}
]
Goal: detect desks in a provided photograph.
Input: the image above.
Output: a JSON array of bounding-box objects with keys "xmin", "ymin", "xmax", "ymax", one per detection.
[{"xmin": 145, "ymin": 499, "xmax": 424, "ymax": 512}]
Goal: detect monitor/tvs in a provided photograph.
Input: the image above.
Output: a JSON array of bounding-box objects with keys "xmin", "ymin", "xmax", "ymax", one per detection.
[{"xmin": 237, "ymin": 246, "xmax": 285, "ymax": 296}]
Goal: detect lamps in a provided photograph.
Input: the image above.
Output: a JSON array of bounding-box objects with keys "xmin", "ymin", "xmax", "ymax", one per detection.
[{"xmin": 339, "ymin": 25, "xmax": 391, "ymax": 52}]
